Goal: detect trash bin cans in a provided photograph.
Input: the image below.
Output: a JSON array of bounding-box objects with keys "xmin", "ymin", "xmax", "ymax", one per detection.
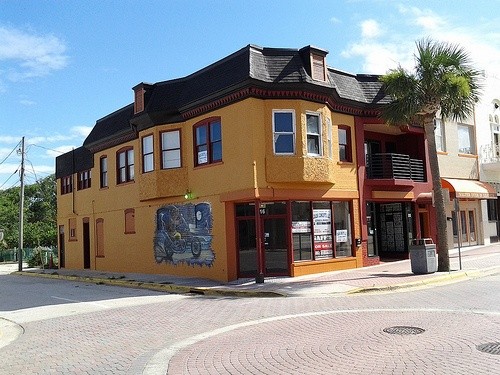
[{"xmin": 408, "ymin": 237, "xmax": 437, "ymax": 274}]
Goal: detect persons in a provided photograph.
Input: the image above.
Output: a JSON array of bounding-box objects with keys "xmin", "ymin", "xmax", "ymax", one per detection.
[{"xmin": 165, "ymin": 204, "xmax": 183, "ymax": 241}]
